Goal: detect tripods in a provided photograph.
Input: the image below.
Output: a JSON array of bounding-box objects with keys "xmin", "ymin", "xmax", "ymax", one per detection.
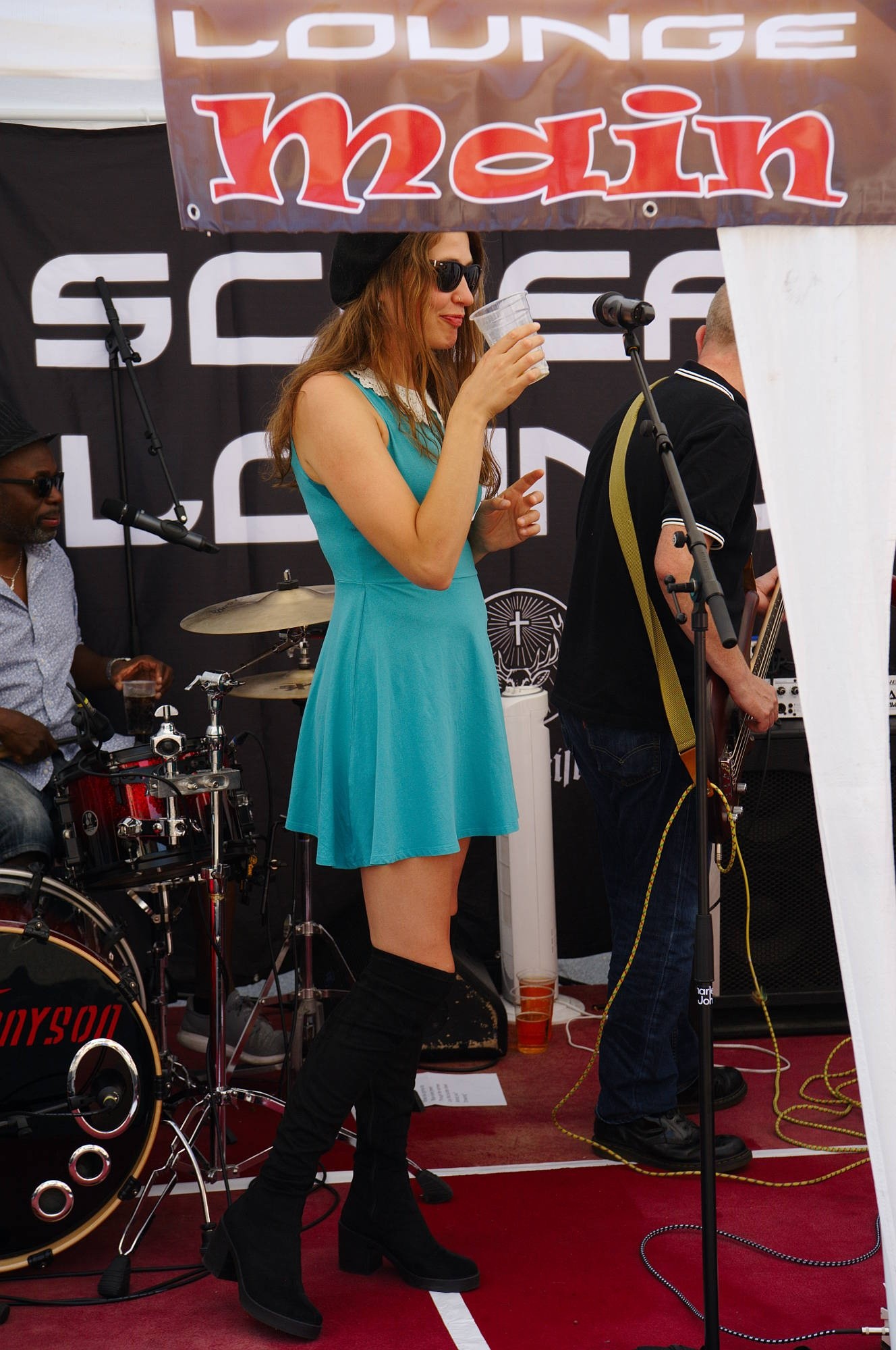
[{"xmin": 97, "ymin": 628, "xmax": 454, "ymax": 1301}]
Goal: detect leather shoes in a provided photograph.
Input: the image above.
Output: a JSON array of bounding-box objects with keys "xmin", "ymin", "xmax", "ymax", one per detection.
[
  {"xmin": 672, "ymin": 1065, "xmax": 748, "ymax": 1115},
  {"xmin": 592, "ymin": 1109, "xmax": 751, "ymax": 1173}
]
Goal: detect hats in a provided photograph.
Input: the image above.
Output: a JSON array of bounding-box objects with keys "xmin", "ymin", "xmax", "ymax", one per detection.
[
  {"xmin": 0, "ymin": 399, "xmax": 58, "ymax": 460},
  {"xmin": 329, "ymin": 230, "xmax": 407, "ymax": 310}
]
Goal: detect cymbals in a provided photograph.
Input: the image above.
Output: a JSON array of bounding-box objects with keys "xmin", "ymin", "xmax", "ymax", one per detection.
[
  {"xmin": 179, "ymin": 585, "xmax": 335, "ymax": 635},
  {"xmin": 226, "ymin": 669, "xmax": 315, "ymax": 699}
]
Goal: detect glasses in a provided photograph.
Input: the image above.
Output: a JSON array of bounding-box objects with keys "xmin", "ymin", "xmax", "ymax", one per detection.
[
  {"xmin": 0, "ymin": 470, "xmax": 65, "ymax": 499},
  {"xmin": 424, "ymin": 260, "xmax": 481, "ymax": 292}
]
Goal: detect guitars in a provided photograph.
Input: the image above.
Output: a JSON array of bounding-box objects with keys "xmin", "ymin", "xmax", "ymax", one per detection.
[{"xmin": 706, "ymin": 578, "xmax": 785, "ymax": 834}]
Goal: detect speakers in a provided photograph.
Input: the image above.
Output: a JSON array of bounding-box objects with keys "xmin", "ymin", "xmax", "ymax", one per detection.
[{"xmin": 712, "ymin": 718, "xmax": 850, "ymax": 1041}]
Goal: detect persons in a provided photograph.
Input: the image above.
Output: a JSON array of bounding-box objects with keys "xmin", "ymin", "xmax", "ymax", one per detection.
[
  {"xmin": 206, "ymin": 232, "xmax": 549, "ymax": 1341},
  {"xmin": 551, "ymin": 281, "xmax": 787, "ymax": 1172},
  {"xmin": 0, "ymin": 425, "xmax": 287, "ymax": 1064}
]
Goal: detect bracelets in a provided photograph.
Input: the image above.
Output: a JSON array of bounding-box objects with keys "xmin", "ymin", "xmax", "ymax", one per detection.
[{"xmin": 107, "ymin": 656, "xmax": 130, "ymax": 678}]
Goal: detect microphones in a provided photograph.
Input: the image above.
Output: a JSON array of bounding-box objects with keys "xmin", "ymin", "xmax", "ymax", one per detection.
[
  {"xmin": 67, "ymin": 687, "xmax": 115, "ymax": 743},
  {"xmin": 100, "ymin": 498, "xmax": 221, "ymax": 555},
  {"xmin": 100, "ymin": 1088, "xmax": 123, "ymax": 1110},
  {"xmin": 594, "ymin": 292, "xmax": 655, "ymax": 330}
]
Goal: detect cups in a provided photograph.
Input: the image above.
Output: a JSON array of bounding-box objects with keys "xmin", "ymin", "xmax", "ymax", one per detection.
[
  {"xmin": 469, "ymin": 290, "xmax": 549, "ymax": 386},
  {"xmin": 509, "ymin": 986, "xmax": 556, "ymax": 1056},
  {"xmin": 121, "ymin": 680, "xmax": 157, "ymax": 735},
  {"xmin": 516, "ymin": 967, "xmax": 558, "ymax": 1041}
]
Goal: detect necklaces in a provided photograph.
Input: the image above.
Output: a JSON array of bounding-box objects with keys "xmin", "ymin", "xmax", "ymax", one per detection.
[{"xmin": 0, "ymin": 551, "xmax": 23, "ymax": 590}]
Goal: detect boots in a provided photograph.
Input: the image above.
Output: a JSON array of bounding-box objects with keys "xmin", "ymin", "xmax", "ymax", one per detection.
[
  {"xmin": 337, "ymin": 1023, "xmax": 480, "ymax": 1293},
  {"xmin": 201, "ymin": 952, "xmax": 456, "ymax": 1339}
]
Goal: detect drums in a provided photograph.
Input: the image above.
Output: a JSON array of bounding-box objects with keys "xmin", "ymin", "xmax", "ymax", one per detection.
[
  {"xmin": 0, "ymin": 867, "xmax": 162, "ymax": 1273},
  {"xmin": 51, "ymin": 737, "xmax": 247, "ymax": 899}
]
